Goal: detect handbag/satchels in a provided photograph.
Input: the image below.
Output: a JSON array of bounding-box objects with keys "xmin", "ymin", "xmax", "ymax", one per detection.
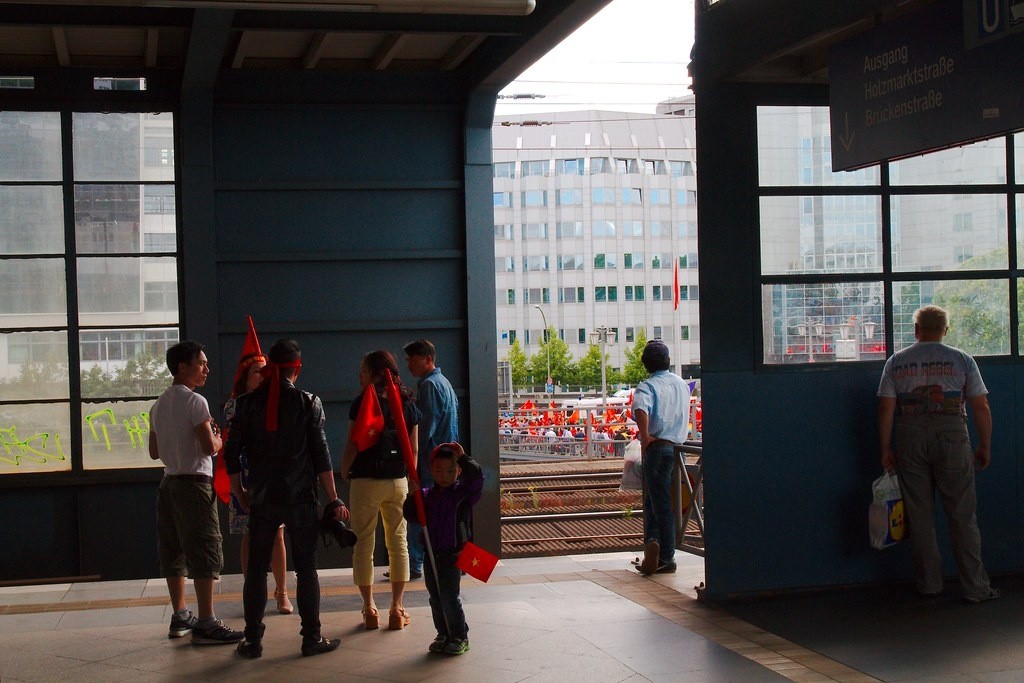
[
  {"xmin": 620, "ymin": 438, "xmax": 643, "ymax": 490},
  {"xmin": 870, "ymin": 466, "xmax": 909, "ymax": 548}
]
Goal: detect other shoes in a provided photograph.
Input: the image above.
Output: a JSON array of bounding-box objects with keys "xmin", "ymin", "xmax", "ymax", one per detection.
[
  {"xmin": 238, "ymin": 641, "xmax": 263, "ymax": 658},
  {"xmin": 301, "ymin": 636, "xmax": 340, "ymax": 656},
  {"xmin": 383, "ymin": 568, "xmax": 422, "ymax": 579}
]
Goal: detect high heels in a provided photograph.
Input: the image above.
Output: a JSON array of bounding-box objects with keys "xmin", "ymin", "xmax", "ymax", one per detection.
[
  {"xmin": 389, "ymin": 606, "xmax": 410, "ymax": 629},
  {"xmin": 361, "ymin": 606, "xmax": 379, "ymax": 629},
  {"xmin": 274, "ymin": 587, "xmax": 294, "ymax": 614}
]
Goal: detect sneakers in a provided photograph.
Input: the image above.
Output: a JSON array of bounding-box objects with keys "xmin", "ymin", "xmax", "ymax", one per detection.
[
  {"xmin": 429, "ymin": 634, "xmax": 469, "ymax": 655},
  {"xmin": 168, "ymin": 609, "xmax": 197, "ymax": 637},
  {"xmin": 191, "ymin": 620, "xmax": 244, "ymax": 644},
  {"xmin": 635, "ymin": 559, "xmax": 676, "ymax": 573},
  {"xmin": 921, "ymin": 583, "xmax": 943, "ymax": 597},
  {"xmin": 968, "ymin": 588, "xmax": 1001, "ymax": 603},
  {"xmin": 641, "ymin": 537, "xmax": 660, "ymax": 574}
]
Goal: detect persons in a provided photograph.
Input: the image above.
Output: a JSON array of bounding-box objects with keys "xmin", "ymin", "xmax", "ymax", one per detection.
[
  {"xmin": 876, "ymin": 306, "xmax": 1003, "ymax": 602},
  {"xmin": 403, "ymin": 440, "xmax": 485, "ymax": 654},
  {"xmin": 223, "ymin": 339, "xmax": 350, "ymax": 659},
  {"xmin": 383, "ymin": 339, "xmax": 459, "ymax": 580},
  {"xmin": 499, "ymin": 420, "xmax": 633, "ymax": 458},
  {"xmin": 341, "ymin": 350, "xmax": 420, "ymax": 629},
  {"xmin": 220, "ymin": 353, "xmax": 293, "ymax": 614},
  {"xmin": 149, "ymin": 341, "xmax": 244, "ymax": 645},
  {"xmin": 633, "ymin": 341, "xmax": 690, "ymax": 574}
]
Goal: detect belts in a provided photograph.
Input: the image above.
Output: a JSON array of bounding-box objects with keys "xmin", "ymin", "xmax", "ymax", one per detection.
[{"xmin": 649, "ymin": 440, "xmax": 673, "ymax": 445}]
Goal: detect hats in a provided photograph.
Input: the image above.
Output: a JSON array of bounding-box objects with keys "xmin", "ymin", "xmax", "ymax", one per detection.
[{"xmin": 430, "ymin": 443, "xmax": 462, "ymax": 461}]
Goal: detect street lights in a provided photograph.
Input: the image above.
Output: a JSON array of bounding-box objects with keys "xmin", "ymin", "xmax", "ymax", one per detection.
[
  {"xmin": 535, "ymin": 305, "xmax": 550, "ymax": 385},
  {"xmin": 838, "ymin": 315, "xmax": 878, "ymax": 361},
  {"xmin": 797, "ymin": 315, "xmax": 824, "ymax": 359},
  {"xmin": 590, "ymin": 325, "xmax": 616, "ymax": 415}
]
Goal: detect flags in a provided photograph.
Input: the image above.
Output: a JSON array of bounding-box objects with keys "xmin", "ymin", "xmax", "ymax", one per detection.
[
  {"xmin": 350, "ymin": 383, "xmax": 384, "ymax": 451},
  {"xmin": 455, "ymin": 542, "xmax": 499, "ymax": 583},
  {"xmin": 674, "ymin": 260, "xmax": 679, "ymax": 311}
]
